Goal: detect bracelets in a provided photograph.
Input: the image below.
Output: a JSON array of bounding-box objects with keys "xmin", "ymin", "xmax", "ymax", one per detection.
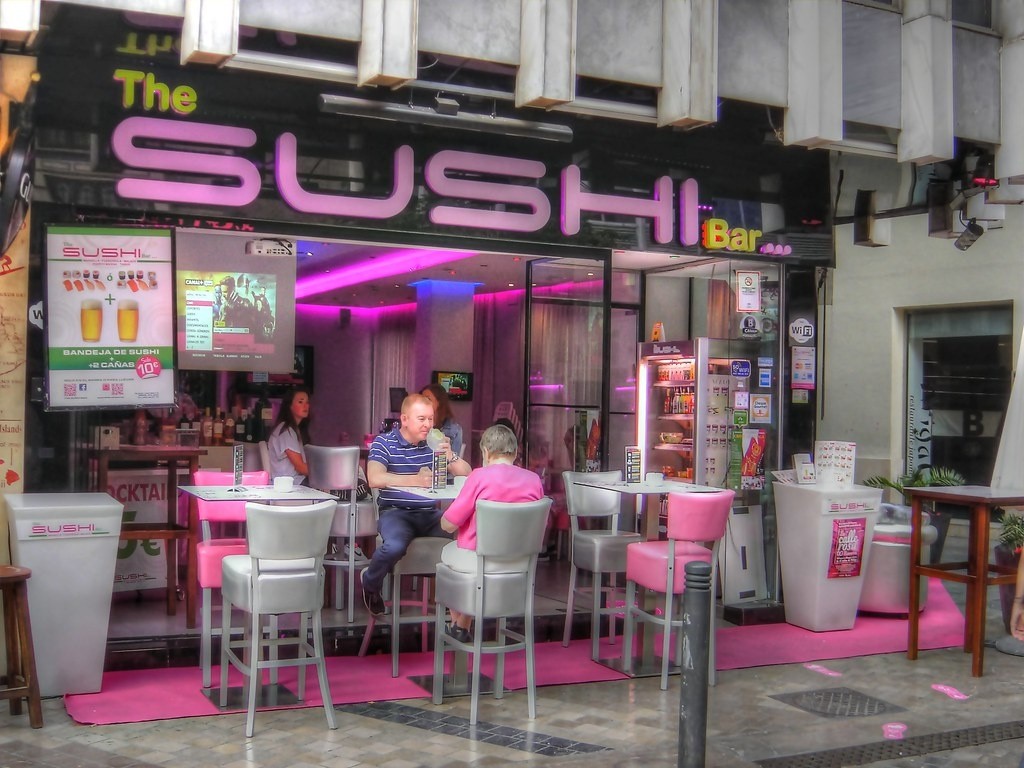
[{"xmin": 448, "ymin": 451, "xmax": 459, "ymax": 465}]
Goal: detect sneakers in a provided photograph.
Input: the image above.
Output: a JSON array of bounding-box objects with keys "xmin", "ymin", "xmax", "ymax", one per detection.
[
  {"xmin": 360, "ymin": 567, "xmax": 386, "ymax": 615},
  {"xmin": 331, "ymin": 543, "xmax": 368, "ymax": 560}
]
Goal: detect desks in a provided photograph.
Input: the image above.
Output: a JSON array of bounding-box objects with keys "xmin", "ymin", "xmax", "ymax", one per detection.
[
  {"xmin": 388, "ymin": 482, "xmax": 512, "ymax": 699},
  {"xmin": 576, "ymin": 480, "xmax": 728, "ymax": 678},
  {"xmin": 772, "ymin": 481, "xmax": 884, "ymax": 632},
  {"xmin": 900, "ymin": 486, "xmax": 1024, "ymax": 678},
  {"xmin": 178, "ymin": 484, "xmax": 338, "ymax": 713},
  {"xmin": 3, "ymin": 493, "xmax": 124, "ymax": 697}
]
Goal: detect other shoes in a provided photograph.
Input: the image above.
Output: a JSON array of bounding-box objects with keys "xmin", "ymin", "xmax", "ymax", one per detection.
[{"xmin": 445, "ymin": 621, "xmax": 472, "ymax": 642}]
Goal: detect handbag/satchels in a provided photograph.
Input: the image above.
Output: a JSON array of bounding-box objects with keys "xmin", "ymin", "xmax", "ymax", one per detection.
[{"xmin": 330, "ymin": 465, "xmax": 369, "ymax": 501}]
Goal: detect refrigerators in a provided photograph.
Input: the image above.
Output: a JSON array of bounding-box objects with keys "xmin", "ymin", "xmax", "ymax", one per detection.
[{"xmin": 635, "ymin": 339, "xmax": 781, "ymax": 541}]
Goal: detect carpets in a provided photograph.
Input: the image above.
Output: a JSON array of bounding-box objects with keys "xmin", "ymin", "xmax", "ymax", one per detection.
[{"xmin": 63, "ymin": 574, "xmax": 965, "ymax": 725}]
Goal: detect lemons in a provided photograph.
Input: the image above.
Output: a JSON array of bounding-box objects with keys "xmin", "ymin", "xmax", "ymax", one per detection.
[{"xmin": 431, "ymin": 429, "xmax": 443, "ymax": 440}]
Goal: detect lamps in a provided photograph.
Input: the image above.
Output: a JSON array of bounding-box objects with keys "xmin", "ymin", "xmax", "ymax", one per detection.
[
  {"xmin": 954, "ymin": 205, "xmax": 984, "ymax": 251},
  {"xmin": 316, "ymin": 87, "xmax": 574, "ymax": 143},
  {"xmin": 972, "ymin": 154, "xmax": 997, "ymax": 186}
]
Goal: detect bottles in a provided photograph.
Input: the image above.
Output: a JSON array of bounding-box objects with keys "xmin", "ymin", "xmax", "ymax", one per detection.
[
  {"xmin": 160, "ymin": 425, "xmax": 177, "ymax": 447},
  {"xmin": 664, "ymin": 388, "xmax": 695, "ymax": 414},
  {"xmin": 133, "ymin": 409, "xmax": 147, "ymax": 445},
  {"xmin": 199, "ymin": 407, "xmax": 213, "ymax": 446},
  {"xmin": 235, "ymin": 415, "xmax": 245, "ymax": 442},
  {"xmin": 244, "ymin": 414, "xmax": 255, "ymax": 443},
  {"xmin": 254, "ymin": 390, "xmax": 272, "ymax": 443},
  {"xmin": 658, "ymin": 363, "xmax": 694, "ymax": 381},
  {"xmin": 213, "ymin": 408, "xmax": 223, "ymax": 446}
]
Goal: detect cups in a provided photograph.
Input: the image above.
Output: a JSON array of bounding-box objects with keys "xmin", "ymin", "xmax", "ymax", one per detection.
[
  {"xmin": 118, "ymin": 300, "xmax": 139, "ymax": 342},
  {"xmin": 80, "ymin": 299, "xmax": 104, "ymax": 342},
  {"xmin": 662, "ymin": 465, "xmax": 669, "ymax": 477},
  {"xmin": 678, "ymin": 470, "xmax": 687, "ymax": 477},
  {"xmin": 667, "ymin": 466, "xmax": 674, "ymax": 477},
  {"xmin": 686, "ymin": 468, "xmax": 693, "ymax": 478}
]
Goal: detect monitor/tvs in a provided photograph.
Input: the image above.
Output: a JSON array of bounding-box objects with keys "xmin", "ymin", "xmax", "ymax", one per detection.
[{"xmin": 433, "ymin": 370, "xmax": 472, "ymax": 400}]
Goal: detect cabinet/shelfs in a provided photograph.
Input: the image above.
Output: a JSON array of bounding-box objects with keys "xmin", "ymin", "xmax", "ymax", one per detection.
[{"xmin": 88, "ymin": 446, "xmax": 207, "ymax": 629}]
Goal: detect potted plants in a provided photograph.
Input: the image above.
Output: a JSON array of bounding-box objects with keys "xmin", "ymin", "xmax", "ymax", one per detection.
[
  {"xmin": 863, "ymin": 466, "xmax": 965, "ymax": 565},
  {"xmin": 994, "ymin": 514, "xmax": 1024, "ymax": 635}
]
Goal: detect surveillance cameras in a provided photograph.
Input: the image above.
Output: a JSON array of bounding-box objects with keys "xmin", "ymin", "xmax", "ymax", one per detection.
[{"xmin": 954, "ymin": 222, "xmax": 982, "ymax": 251}]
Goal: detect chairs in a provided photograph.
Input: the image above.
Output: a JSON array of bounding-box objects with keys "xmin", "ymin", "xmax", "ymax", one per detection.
[{"xmin": 472, "ymin": 401, "xmax": 523, "ymax": 443}]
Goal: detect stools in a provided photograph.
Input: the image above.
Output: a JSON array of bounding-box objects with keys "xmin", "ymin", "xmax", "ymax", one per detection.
[
  {"xmin": 560, "ymin": 470, "xmax": 736, "ymax": 689},
  {"xmin": 195, "ymin": 470, "xmax": 339, "ymax": 736},
  {"xmin": 259, "ymin": 440, "xmax": 272, "ymax": 482},
  {"xmin": 0, "ymin": 565, "xmax": 43, "ymax": 730},
  {"xmin": 305, "ymin": 444, "xmax": 553, "ymax": 719}
]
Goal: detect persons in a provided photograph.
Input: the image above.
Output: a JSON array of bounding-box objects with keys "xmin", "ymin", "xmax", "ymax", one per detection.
[
  {"xmin": 213, "ymin": 272, "xmax": 276, "ymax": 342},
  {"xmin": 146, "ymin": 390, "xmax": 199, "ymax": 423},
  {"xmin": 439, "ymin": 425, "xmax": 546, "ymax": 643},
  {"xmin": 418, "ymin": 383, "xmax": 463, "ymax": 484},
  {"xmin": 538, "ymin": 426, "xmax": 587, "ymax": 562},
  {"xmin": 267, "ymin": 385, "xmax": 369, "ymax": 561},
  {"xmin": 360, "ymin": 392, "xmax": 474, "ymax": 616}
]
